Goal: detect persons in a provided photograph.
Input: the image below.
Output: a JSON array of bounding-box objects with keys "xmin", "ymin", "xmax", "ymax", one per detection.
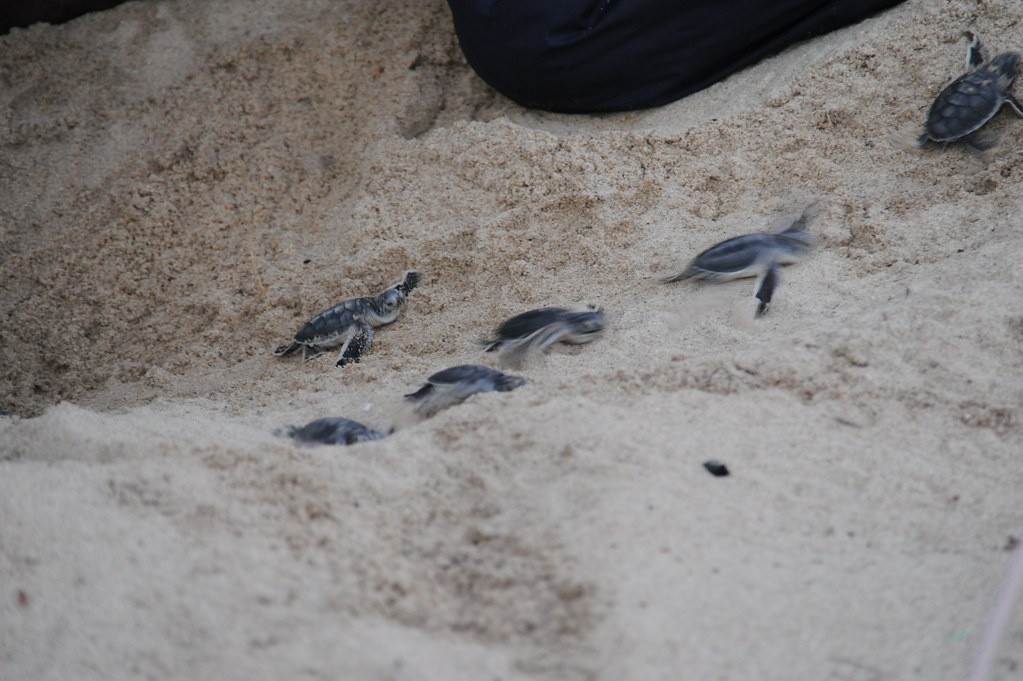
[{"xmin": 447, "ymin": 0, "xmax": 905, "ymax": 117}]
